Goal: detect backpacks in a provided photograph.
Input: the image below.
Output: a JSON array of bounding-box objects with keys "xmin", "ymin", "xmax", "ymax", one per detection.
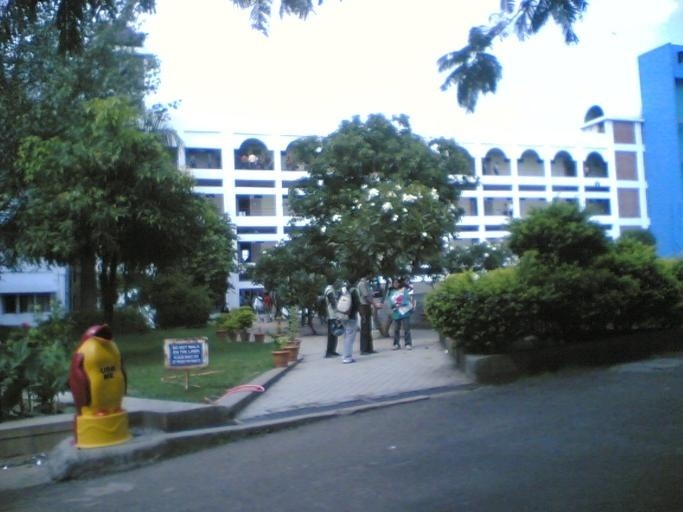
[{"xmin": 335, "ymin": 287, "xmax": 355, "ymax": 316}]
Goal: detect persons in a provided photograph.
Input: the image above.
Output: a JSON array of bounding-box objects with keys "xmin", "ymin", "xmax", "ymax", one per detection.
[
  {"xmin": 253, "ymin": 293, "xmax": 265, "ymax": 321},
  {"xmin": 386, "ymin": 279, "xmax": 401, "ymax": 334},
  {"xmin": 264, "ymin": 291, "xmax": 273, "ymax": 323},
  {"xmin": 337, "ymin": 277, "xmax": 364, "ymax": 365},
  {"xmin": 356, "ymin": 274, "xmax": 386, "ymax": 354},
  {"xmin": 387, "ymin": 279, "xmax": 417, "ymax": 348},
  {"xmin": 324, "ymin": 274, "xmax": 342, "ymax": 356}
]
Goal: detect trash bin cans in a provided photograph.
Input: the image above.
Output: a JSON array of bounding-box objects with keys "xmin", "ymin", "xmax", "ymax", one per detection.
[{"xmin": 69, "ymin": 324, "xmax": 130, "ymax": 448}]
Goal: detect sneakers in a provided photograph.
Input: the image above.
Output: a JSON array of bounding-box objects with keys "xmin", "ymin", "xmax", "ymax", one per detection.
[
  {"xmin": 326, "ymin": 351, "xmax": 377, "ymax": 363},
  {"xmin": 392, "ymin": 345, "xmax": 398, "ymax": 350},
  {"xmin": 405, "ymin": 344, "xmax": 411, "ymax": 350}
]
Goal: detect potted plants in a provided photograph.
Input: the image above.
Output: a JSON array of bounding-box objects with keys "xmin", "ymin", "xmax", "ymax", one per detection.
[{"xmin": 211, "ymin": 303, "xmax": 302, "ymax": 367}]
